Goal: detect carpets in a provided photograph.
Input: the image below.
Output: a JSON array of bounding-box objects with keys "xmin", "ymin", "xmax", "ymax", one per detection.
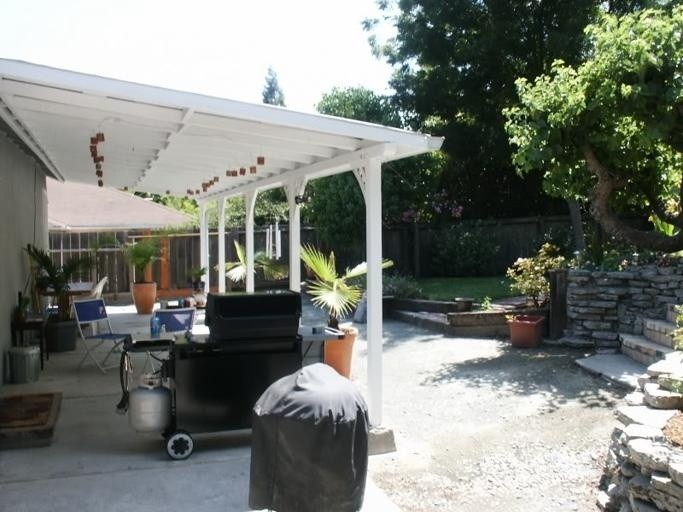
[{"xmin": 1, "ymin": 389, "xmax": 65, "ymax": 454}]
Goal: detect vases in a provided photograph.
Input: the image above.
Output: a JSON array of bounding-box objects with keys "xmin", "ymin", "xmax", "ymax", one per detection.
[{"xmin": 503, "ymin": 313, "xmax": 545, "ymax": 349}]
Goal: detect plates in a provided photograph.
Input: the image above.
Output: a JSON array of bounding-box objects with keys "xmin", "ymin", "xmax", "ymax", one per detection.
[
  {"xmin": 167, "ymin": 305, "xmax": 181, "ymax": 309},
  {"xmin": 196, "ymin": 305, "xmax": 204, "ymax": 309}
]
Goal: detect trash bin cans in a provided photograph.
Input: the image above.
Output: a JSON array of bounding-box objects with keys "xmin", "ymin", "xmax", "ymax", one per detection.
[{"xmin": 9, "ymin": 346, "xmax": 40, "ymax": 383}]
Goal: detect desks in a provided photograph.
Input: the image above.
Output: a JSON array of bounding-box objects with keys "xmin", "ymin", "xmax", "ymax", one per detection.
[{"xmin": 10, "ymin": 313, "xmax": 52, "ymax": 370}]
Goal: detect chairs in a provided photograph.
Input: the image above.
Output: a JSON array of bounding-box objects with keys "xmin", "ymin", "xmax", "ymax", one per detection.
[
  {"xmin": 143, "ymin": 307, "xmax": 193, "ymax": 372},
  {"xmin": 70, "ymin": 296, "xmax": 132, "ymax": 376},
  {"xmin": 67, "ymin": 275, "xmax": 109, "ymax": 337}
]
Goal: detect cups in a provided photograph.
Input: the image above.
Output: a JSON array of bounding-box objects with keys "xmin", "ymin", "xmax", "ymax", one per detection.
[
  {"xmin": 185, "ymin": 300, "xmax": 190, "ymax": 307},
  {"xmin": 159, "ymin": 299, "xmax": 169, "ymax": 309},
  {"xmin": 178, "ymin": 298, "xmax": 184, "ymax": 307}
]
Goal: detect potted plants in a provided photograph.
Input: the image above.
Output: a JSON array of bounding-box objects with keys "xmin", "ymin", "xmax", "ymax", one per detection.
[
  {"xmin": 123, "ymin": 229, "xmax": 165, "ymax": 316},
  {"xmin": 21, "ymin": 242, "xmax": 103, "ymax": 353},
  {"xmin": 298, "ymin": 238, "xmax": 392, "ymax": 378}
]
